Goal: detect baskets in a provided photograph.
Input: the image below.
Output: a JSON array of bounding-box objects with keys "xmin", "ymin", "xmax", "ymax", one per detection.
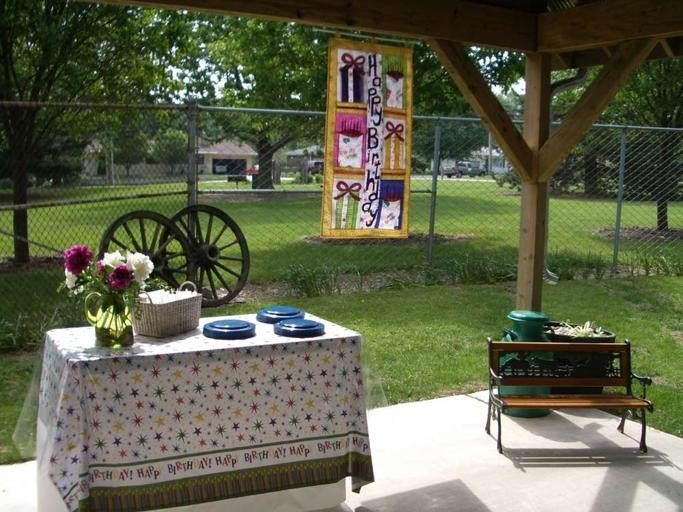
[{"xmin": 131, "ymin": 280, "xmax": 204, "ymax": 339}]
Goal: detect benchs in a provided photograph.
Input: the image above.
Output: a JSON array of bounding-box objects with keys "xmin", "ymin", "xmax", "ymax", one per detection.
[{"xmin": 485, "ymin": 337, "xmax": 653, "ymax": 455}]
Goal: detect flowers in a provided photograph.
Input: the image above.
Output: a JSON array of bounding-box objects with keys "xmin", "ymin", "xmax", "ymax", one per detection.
[
  {"xmin": 546, "ymin": 319, "xmax": 605, "ymax": 337},
  {"xmin": 56, "ymin": 244, "xmax": 175, "ymax": 328}
]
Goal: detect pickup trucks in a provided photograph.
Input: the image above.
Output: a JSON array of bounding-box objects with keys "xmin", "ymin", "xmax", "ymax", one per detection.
[{"xmin": 443, "ymin": 161, "xmax": 482, "ymax": 177}]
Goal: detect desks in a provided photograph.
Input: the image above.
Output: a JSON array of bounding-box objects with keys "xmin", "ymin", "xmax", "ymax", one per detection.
[{"xmin": 12, "ymin": 312, "xmax": 374, "ymax": 512}]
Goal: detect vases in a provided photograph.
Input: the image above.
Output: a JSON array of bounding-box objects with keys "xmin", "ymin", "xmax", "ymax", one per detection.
[{"xmin": 84, "ymin": 293, "xmax": 134, "ymax": 349}]
